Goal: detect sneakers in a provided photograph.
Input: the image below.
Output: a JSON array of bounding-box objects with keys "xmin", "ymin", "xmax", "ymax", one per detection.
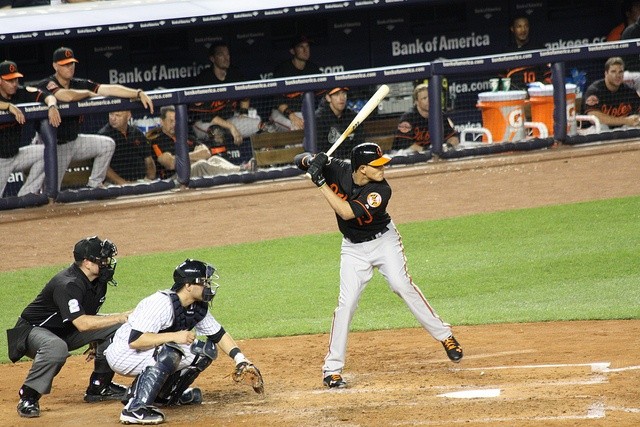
[
  {"xmin": 121, "ymin": 397, "xmax": 164, "ymax": 424},
  {"xmin": 246, "ymin": 158, "xmax": 258, "ymax": 172},
  {"xmin": 85, "ymin": 382, "xmax": 128, "ymax": 403},
  {"xmin": 18, "ymin": 398, "xmax": 40, "ymax": 417},
  {"xmin": 179, "ymin": 388, "xmax": 200, "ymax": 403},
  {"xmin": 86, "ymin": 181, "xmax": 105, "ymax": 189},
  {"xmin": 323, "ymin": 376, "xmax": 347, "ymax": 389},
  {"xmin": 443, "ymin": 336, "xmax": 462, "ymax": 361}
]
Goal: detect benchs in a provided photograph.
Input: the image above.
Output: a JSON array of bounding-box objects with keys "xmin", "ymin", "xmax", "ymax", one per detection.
[
  {"xmin": 23, "ymin": 111, "xmax": 407, "ymax": 191},
  {"xmin": 523, "ymin": 97, "xmax": 588, "ymax": 135}
]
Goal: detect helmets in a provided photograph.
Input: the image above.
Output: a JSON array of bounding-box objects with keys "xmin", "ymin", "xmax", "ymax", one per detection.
[
  {"xmin": 171, "ymin": 260, "xmax": 219, "ymax": 309},
  {"xmin": 72, "ymin": 237, "xmax": 118, "ymax": 288}
]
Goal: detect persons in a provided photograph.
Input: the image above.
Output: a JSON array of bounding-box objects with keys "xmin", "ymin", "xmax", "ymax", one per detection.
[
  {"xmin": 6, "ymin": 236, "xmax": 128, "ymax": 416},
  {"xmin": 152, "ymin": 106, "xmax": 257, "ymax": 181},
  {"xmin": 268, "ymin": 32, "xmax": 331, "ymax": 132},
  {"xmin": 103, "ymin": 258, "xmax": 266, "ymax": 423},
  {"xmin": 393, "ymin": 84, "xmax": 461, "ymax": 149},
  {"xmin": 607, "ymin": 2, "xmax": 640, "ymax": 42},
  {"xmin": 0, "ymin": 59, "xmax": 62, "ymax": 198},
  {"xmin": 87, "ymin": 109, "xmax": 156, "ymax": 184},
  {"xmin": 302, "ymin": 88, "xmax": 365, "ymax": 159},
  {"xmin": 580, "ymin": 56, "xmax": 640, "ymax": 131},
  {"xmin": 496, "ymin": 17, "xmax": 551, "ymax": 90},
  {"xmin": 294, "ymin": 142, "xmax": 464, "ymax": 389},
  {"xmin": 33, "ymin": 46, "xmax": 155, "ymax": 192},
  {"xmin": 186, "ymin": 41, "xmax": 261, "ymax": 145}
]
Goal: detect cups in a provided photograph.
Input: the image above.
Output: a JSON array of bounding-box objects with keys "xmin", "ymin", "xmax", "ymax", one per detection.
[
  {"xmin": 490, "ymin": 77, "xmax": 500, "ymax": 94},
  {"xmin": 504, "ymin": 76, "xmax": 511, "ymax": 90}
]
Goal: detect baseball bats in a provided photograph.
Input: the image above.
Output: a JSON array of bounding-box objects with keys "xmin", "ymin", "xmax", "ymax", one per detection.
[{"xmin": 305, "ymin": 84, "xmax": 390, "ymax": 180}]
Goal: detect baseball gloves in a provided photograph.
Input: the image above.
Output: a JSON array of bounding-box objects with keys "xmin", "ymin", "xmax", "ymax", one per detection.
[{"xmin": 234, "ymin": 362, "xmax": 264, "ymax": 392}]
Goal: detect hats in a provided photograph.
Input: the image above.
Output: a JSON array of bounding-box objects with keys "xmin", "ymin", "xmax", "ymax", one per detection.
[
  {"xmin": 328, "ymin": 85, "xmax": 351, "ymax": 96},
  {"xmin": 0, "ymin": 61, "xmax": 24, "ymax": 81},
  {"xmin": 351, "ymin": 142, "xmax": 392, "ymax": 167},
  {"xmin": 53, "ymin": 47, "xmax": 79, "ymax": 65}
]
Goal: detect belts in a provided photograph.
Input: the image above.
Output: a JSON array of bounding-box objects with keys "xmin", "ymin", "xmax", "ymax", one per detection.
[{"xmin": 344, "ymin": 217, "xmax": 392, "ymax": 244}]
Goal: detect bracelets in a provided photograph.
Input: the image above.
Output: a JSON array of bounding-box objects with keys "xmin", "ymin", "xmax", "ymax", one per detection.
[
  {"xmin": 7, "ymin": 103, "xmax": 11, "ymax": 111},
  {"xmin": 48, "ymin": 105, "xmax": 58, "ymax": 109},
  {"xmin": 136, "ymin": 88, "xmax": 142, "ymax": 98}
]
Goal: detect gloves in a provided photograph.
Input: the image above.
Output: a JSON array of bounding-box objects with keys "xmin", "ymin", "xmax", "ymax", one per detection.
[
  {"xmin": 308, "ymin": 152, "xmax": 328, "ymax": 166},
  {"xmin": 308, "ymin": 164, "xmax": 325, "ymax": 188}
]
